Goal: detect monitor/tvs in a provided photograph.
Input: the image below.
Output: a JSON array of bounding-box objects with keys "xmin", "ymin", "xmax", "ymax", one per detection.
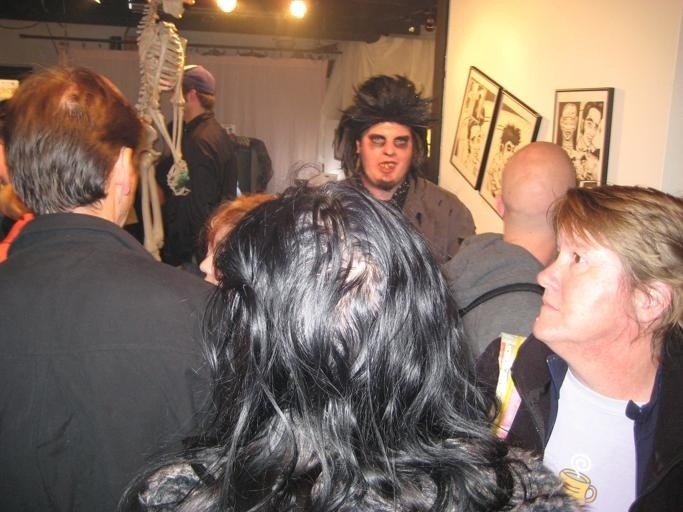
[{"xmin": 231, "ymin": 136, "xmax": 272, "ymax": 197}]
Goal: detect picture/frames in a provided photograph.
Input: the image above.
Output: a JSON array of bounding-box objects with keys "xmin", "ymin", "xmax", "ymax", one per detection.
[
  {"xmin": 449, "ymin": 64, "xmax": 503, "ymax": 192},
  {"xmin": 475, "ymin": 84, "xmax": 543, "ymax": 220},
  {"xmin": 549, "ymin": 84, "xmax": 616, "ymax": 191}
]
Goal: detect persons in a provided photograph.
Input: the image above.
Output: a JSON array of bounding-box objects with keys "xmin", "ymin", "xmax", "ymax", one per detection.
[
  {"xmin": 0, "ymin": 99, "xmax": 30, "ymax": 248},
  {"xmin": 195, "ymin": 193, "xmax": 280, "ymax": 286},
  {"xmin": 328, "ymin": 68, "xmax": 477, "ymax": 269},
  {"xmin": 470, "ymin": 185, "xmax": 681, "ymax": 512},
  {"xmin": 121, "ymin": 60, "xmax": 239, "ymax": 272},
  {"xmin": 108, "ymin": 176, "xmax": 586, "ymax": 512},
  {"xmin": 433, "ymin": 138, "xmax": 579, "ymax": 369},
  {"xmin": 0, "ymin": 62, "xmax": 221, "ymax": 512}
]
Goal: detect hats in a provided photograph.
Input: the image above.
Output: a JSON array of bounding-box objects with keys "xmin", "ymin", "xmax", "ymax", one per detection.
[{"xmin": 183, "ymin": 64, "xmax": 216, "ymax": 95}]
